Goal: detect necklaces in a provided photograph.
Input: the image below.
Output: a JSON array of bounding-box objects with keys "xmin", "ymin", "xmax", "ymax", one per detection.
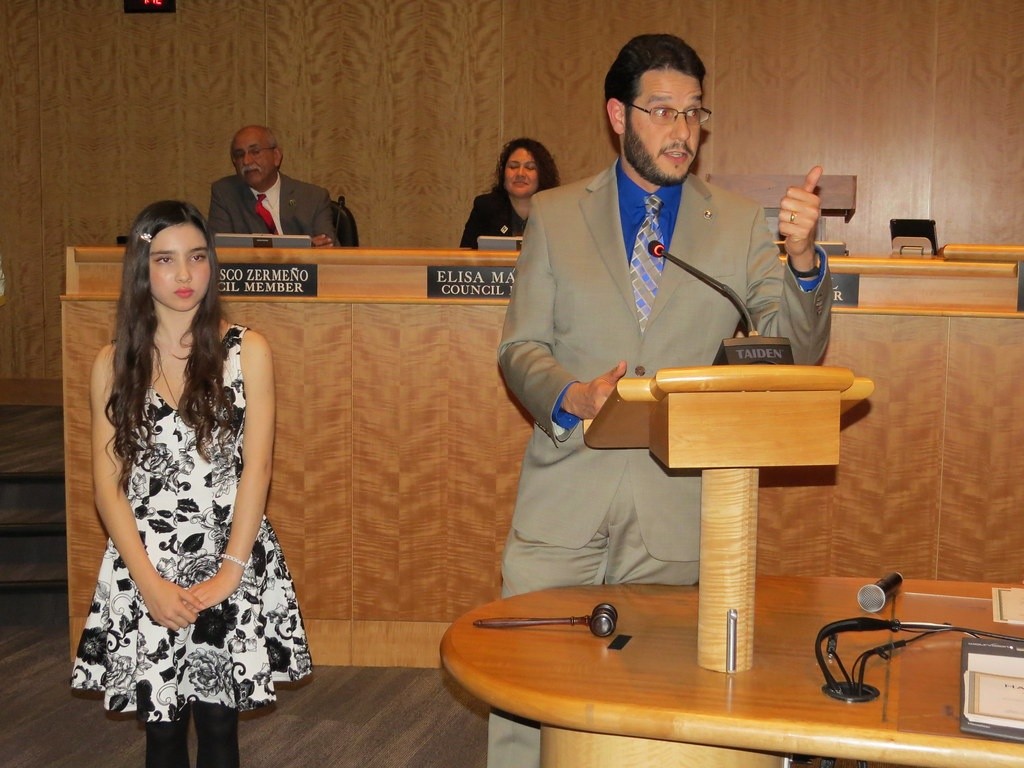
[{"xmin": 159, "ymin": 361, "xmax": 178, "ymax": 409}]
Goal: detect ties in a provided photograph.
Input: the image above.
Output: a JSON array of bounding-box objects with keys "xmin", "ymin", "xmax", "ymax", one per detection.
[
  {"xmin": 255, "ymin": 194, "xmax": 278, "ymax": 235},
  {"xmin": 630, "ymin": 195, "xmax": 664, "ymax": 335}
]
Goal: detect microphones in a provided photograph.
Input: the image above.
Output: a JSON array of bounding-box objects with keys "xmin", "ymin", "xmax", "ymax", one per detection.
[
  {"xmin": 332, "ymin": 195, "xmax": 346, "ymax": 246},
  {"xmin": 856, "ymin": 571, "xmax": 904, "ymax": 613},
  {"xmin": 648, "ymin": 240, "xmax": 796, "ymax": 367}
]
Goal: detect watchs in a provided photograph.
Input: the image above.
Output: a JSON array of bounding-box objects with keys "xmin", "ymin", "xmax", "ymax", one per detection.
[{"xmin": 787, "ymin": 252, "xmax": 821, "ymax": 278}]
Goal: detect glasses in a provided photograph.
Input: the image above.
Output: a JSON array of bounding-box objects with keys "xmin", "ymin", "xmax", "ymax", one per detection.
[
  {"xmin": 624, "ymin": 101, "xmax": 712, "ymax": 126},
  {"xmin": 232, "ymin": 146, "xmax": 274, "ymax": 160}
]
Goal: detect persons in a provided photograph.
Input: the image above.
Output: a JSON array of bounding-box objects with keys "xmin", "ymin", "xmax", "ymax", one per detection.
[
  {"xmin": 486, "ymin": 35, "xmax": 833, "ymax": 767},
  {"xmin": 459, "ymin": 135, "xmax": 563, "ymax": 251},
  {"xmin": 206, "ymin": 124, "xmax": 342, "ymax": 246},
  {"xmin": 68, "ymin": 199, "xmax": 311, "ymax": 768}
]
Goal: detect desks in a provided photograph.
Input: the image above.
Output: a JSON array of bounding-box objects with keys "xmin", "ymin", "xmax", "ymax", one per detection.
[{"xmin": 438, "ymin": 576, "xmax": 1024, "ymax": 768}]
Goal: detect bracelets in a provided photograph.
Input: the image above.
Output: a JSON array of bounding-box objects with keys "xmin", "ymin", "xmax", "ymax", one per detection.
[{"xmin": 221, "ymin": 554, "xmax": 247, "ymax": 568}]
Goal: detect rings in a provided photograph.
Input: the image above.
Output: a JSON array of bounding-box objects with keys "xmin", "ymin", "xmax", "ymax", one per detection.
[{"xmin": 789, "ymin": 211, "xmax": 796, "ymax": 223}]
